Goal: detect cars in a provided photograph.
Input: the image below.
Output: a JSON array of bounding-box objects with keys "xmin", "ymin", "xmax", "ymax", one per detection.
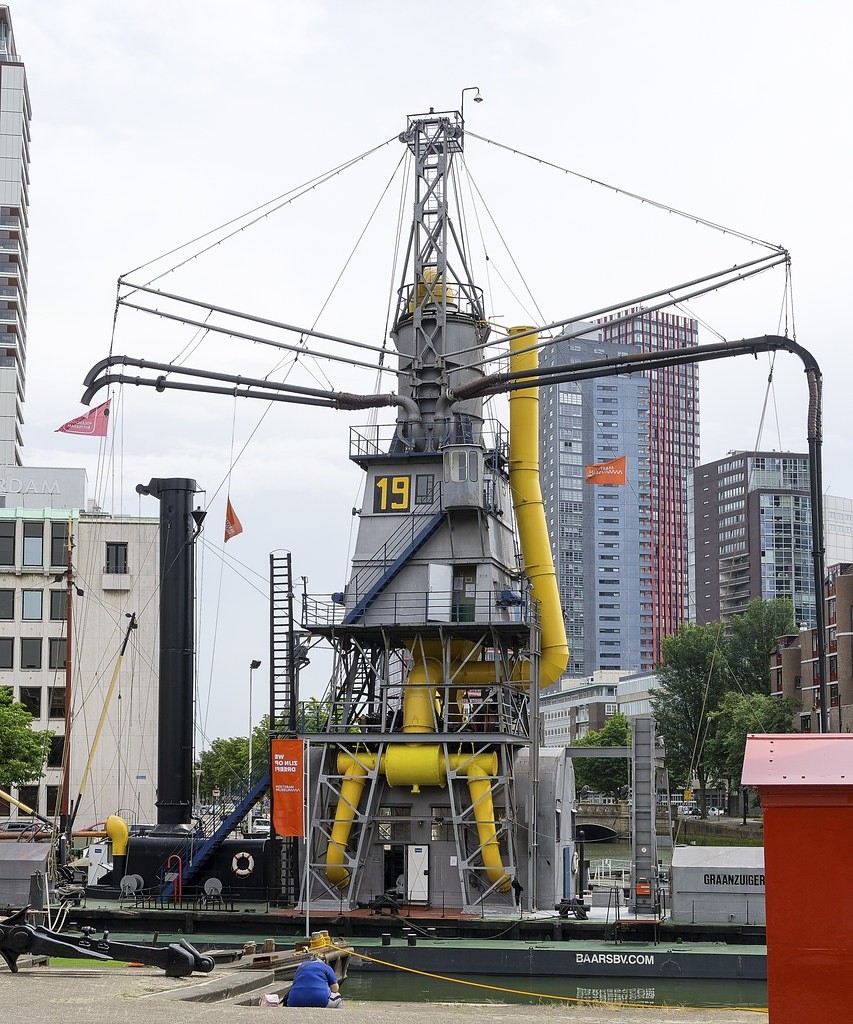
[
  {"xmin": 616, "ymin": 799, "xmax": 726, "ymax": 817},
  {"xmin": 192, "ymin": 795, "xmax": 269, "ymax": 839}
]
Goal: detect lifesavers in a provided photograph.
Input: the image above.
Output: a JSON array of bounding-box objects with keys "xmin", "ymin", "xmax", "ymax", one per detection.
[
  {"xmin": 232, "ymin": 851, "xmax": 255, "ymax": 875},
  {"xmin": 571, "ymin": 851, "xmax": 580, "ymax": 875}
]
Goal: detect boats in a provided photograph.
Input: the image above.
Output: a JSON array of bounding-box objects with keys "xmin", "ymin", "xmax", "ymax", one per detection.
[{"xmin": 0, "ymin": 78, "xmax": 837, "ymax": 981}]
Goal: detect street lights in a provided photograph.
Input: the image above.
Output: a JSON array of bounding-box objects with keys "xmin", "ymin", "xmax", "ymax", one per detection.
[{"xmin": 248, "ymin": 658, "xmax": 261, "ymax": 835}]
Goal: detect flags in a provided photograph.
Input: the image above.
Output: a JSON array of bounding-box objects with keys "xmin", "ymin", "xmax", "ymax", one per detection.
[
  {"xmin": 585, "ymin": 455, "xmax": 628, "ymax": 485},
  {"xmin": 54, "ymin": 398, "xmax": 110, "ymax": 437},
  {"xmin": 225, "ymin": 496, "xmax": 243, "ymax": 543}
]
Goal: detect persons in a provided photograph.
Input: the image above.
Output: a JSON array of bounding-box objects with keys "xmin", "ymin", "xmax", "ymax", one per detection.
[{"xmin": 280, "ymin": 952, "xmax": 342, "ymax": 1009}]
